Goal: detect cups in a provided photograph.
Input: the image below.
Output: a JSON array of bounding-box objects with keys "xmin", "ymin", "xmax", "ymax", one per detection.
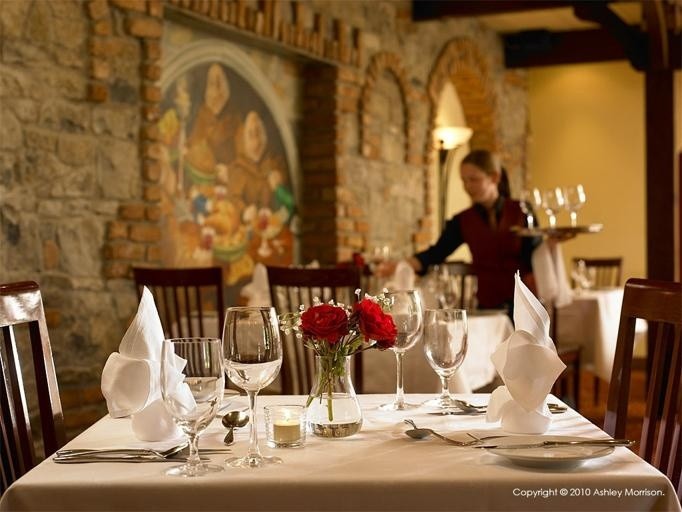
[{"xmin": 262, "ymin": 404, "xmax": 308, "ymax": 450}]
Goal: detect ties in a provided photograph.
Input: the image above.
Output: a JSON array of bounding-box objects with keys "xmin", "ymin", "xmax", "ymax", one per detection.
[{"xmin": 488, "ymin": 208, "xmax": 497, "ymax": 229}]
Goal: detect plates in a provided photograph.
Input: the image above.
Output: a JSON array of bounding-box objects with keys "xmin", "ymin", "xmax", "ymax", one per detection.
[{"xmin": 479, "ymin": 433, "xmax": 616, "ymax": 469}]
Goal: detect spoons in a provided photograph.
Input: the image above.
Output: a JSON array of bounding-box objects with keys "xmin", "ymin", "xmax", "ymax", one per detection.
[
  {"xmin": 54, "ymin": 442, "xmax": 191, "ymax": 458},
  {"xmin": 403, "ymin": 419, "xmax": 435, "ymax": 441},
  {"xmin": 451, "ymin": 395, "xmax": 569, "ymax": 415},
  {"xmin": 220, "ymin": 410, "xmax": 249, "ymax": 446}
]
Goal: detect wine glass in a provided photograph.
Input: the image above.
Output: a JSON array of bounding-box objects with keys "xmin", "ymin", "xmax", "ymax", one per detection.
[
  {"xmin": 159, "ymin": 305, "xmax": 283, "ymax": 478},
  {"xmin": 364, "ymin": 241, "xmax": 390, "ymax": 293},
  {"xmin": 376, "ymin": 291, "xmax": 474, "ymax": 416},
  {"xmin": 514, "ymin": 182, "xmax": 588, "ymax": 229}
]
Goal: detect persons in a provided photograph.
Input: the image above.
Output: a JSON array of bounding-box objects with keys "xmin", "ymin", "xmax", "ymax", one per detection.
[{"xmin": 372, "ymin": 148, "xmax": 577, "ymax": 330}]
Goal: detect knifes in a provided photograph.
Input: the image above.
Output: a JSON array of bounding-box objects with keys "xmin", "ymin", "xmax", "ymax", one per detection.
[{"xmin": 470, "ymin": 439, "xmax": 636, "ymax": 451}]
